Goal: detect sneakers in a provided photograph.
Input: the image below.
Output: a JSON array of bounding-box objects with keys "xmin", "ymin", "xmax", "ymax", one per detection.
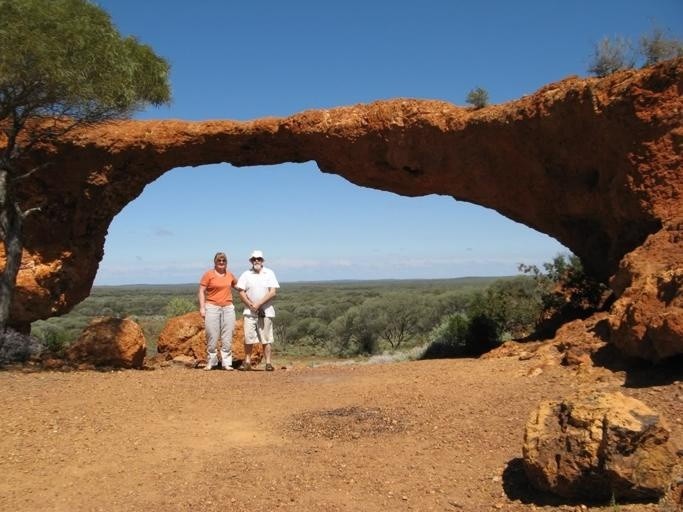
[
  {"xmin": 239, "ymin": 361, "xmax": 251, "ymax": 370},
  {"xmin": 222, "ymin": 366, "xmax": 234, "ymax": 370},
  {"xmin": 266, "ymin": 364, "xmax": 274, "ymax": 370},
  {"xmin": 203, "ymin": 363, "xmax": 218, "ymax": 371}
]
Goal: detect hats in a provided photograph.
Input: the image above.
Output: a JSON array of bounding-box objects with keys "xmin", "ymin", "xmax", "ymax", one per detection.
[{"xmin": 249, "ymin": 251, "xmax": 264, "ymax": 264}]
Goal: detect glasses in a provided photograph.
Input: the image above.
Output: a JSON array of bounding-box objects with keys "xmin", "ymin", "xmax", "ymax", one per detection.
[{"xmin": 253, "ymin": 259, "xmax": 261, "ymax": 261}]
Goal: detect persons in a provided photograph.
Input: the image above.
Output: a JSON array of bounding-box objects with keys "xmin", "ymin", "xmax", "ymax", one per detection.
[
  {"xmin": 198, "ymin": 252, "xmax": 238, "ymax": 371},
  {"xmin": 235, "ymin": 250, "xmax": 281, "ymax": 371}
]
